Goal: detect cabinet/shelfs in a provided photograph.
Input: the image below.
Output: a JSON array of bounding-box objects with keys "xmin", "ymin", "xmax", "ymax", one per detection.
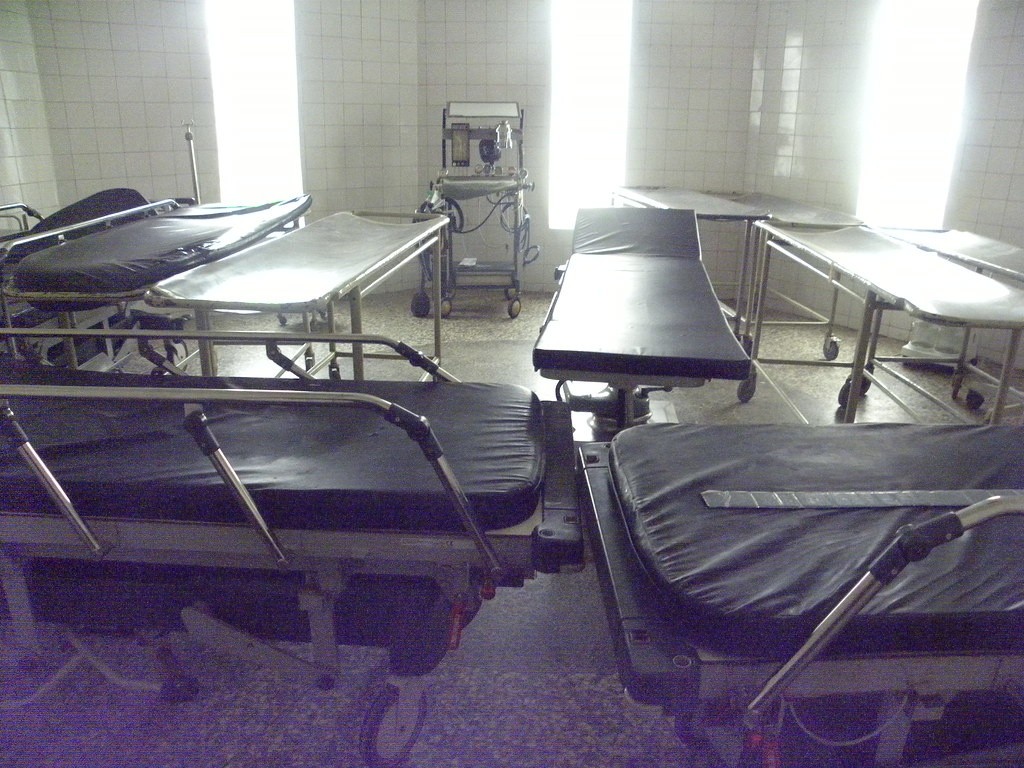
[{"xmin": 410, "ymin": 101, "xmax": 540, "ymax": 319}]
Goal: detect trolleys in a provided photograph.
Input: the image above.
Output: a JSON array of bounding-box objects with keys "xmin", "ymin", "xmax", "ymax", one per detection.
[{"xmin": 412, "ymin": 98, "xmax": 539, "ymax": 320}]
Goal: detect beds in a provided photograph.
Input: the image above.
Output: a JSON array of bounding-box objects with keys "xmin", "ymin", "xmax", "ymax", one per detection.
[{"xmin": 0, "ymin": 186, "xmax": 1024, "ymax": 768}]
